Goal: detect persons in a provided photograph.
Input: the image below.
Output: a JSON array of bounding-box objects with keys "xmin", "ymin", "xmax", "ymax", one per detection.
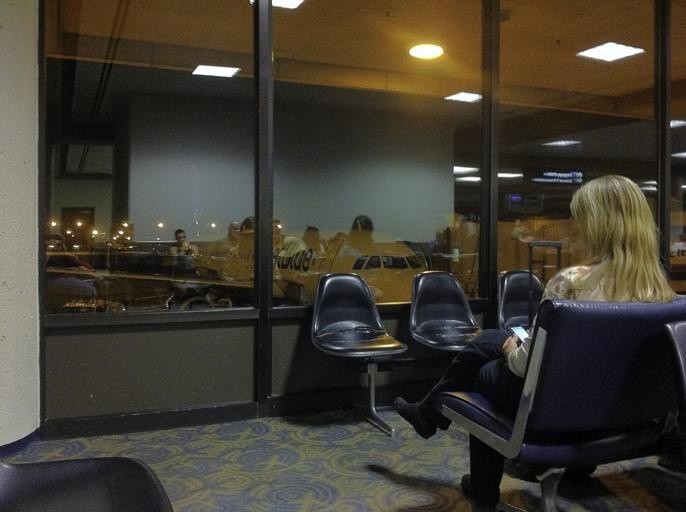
[
  {"xmin": 169, "ymin": 229, "xmax": 202, "ymax": 279},
  {"xmin": 428, "ymin": 230, "xmax": 444, "ymax": 253},
  {"xmin": 208, "ymin": 216, "xmax": 375, "ymax": 305},
  {"xmin": 391, "ymin": 175, "xmax": 681, "ymax": 510},
  {"xmin": 67, "ymin": 254, "xmax": 95, "ymax": 273}
]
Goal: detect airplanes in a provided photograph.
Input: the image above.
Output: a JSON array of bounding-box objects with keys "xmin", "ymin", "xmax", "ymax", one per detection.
[{"xmin": 45, "ymin": 226, "xmax": 429, "ymax": 305}]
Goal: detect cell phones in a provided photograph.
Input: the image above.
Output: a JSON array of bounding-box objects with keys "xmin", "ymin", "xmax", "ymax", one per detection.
[{"xmin": 509, "ymin": 322, "xmax": 530, "ymax": 343}]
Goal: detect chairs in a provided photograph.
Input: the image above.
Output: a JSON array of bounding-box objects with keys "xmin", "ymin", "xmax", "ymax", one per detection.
[
  {"xmin": 408, "ymin": 270, "xmax": 485, "ymax": 390},
  {"xmin": 311, "ymin": 272, "xmax": 408, "ymax": 439},
  {"xmin": 499, "ymin": 271, "xmax": 545, "ymax": 336},
  {"xmin": 434, "ymin": 295, "xmax": 685, "ymax": 512}
]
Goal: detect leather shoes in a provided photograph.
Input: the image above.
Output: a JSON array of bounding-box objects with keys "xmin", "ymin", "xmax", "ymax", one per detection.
[
  {"xmin": 462, "ymin": 474, "xmax": 493, "ymax": 512},
  {"xmin": 395, "ymin": 396, "xmax": 437, "ymax": 439}
]
